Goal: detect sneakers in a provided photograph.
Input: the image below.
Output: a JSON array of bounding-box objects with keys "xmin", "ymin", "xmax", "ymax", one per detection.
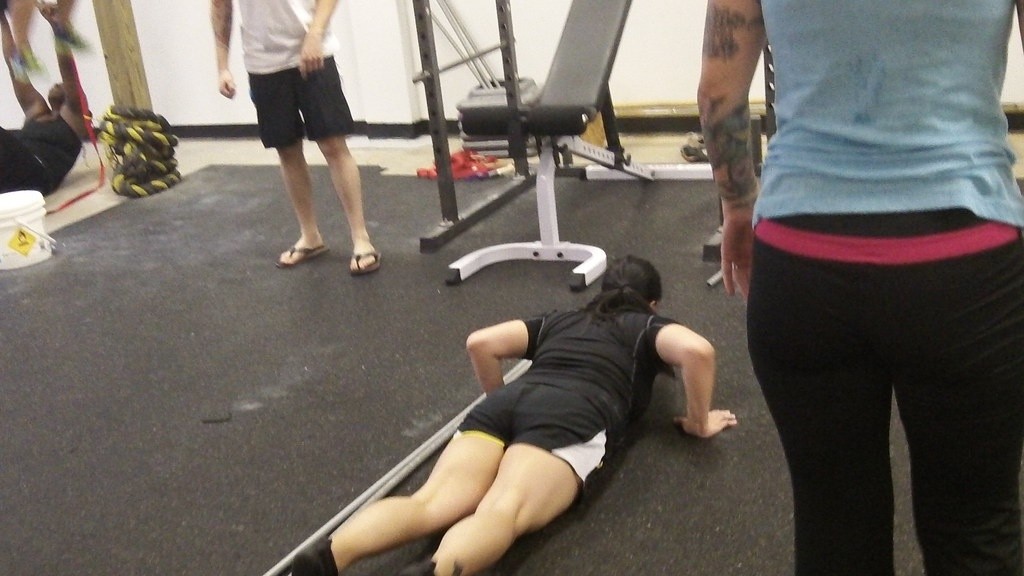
[{"xmin": 293, "ymin": 542, "xmax": 338, "ymax": 575}]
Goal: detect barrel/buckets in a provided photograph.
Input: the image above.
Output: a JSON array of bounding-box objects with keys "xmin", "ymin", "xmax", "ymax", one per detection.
[{"xmin": 0, "ymin": 191, "xmax": 57, "ymax": 270}]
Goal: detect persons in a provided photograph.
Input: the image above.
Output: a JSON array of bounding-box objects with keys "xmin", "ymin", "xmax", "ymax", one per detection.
[
  {"xmin": 699, "ymin": 0, "xmax": 1023, "ymax": 575},
  {"xmin": 1, "ymin": 3, "xmax": 93, "ymax": 199},
  {"xmin": 291, "ymin": 254, "xmax": 738, "ymax": 576},
  {"xmin": 210, "ymin": 2, "xmax": 381, "ymax": 277},
  {"xmin": 1, "ymin": 0, "xmax": 89, "ymax": 71}
]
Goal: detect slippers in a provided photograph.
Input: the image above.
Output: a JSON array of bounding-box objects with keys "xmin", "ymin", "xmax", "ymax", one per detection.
[
  {"xmin": 351, "ymin": 251, "xmax": 380, "ymax": 273},
  {"xmin": 274, "ymin": 245, "xmax": 330, "ymax": 268}
]
